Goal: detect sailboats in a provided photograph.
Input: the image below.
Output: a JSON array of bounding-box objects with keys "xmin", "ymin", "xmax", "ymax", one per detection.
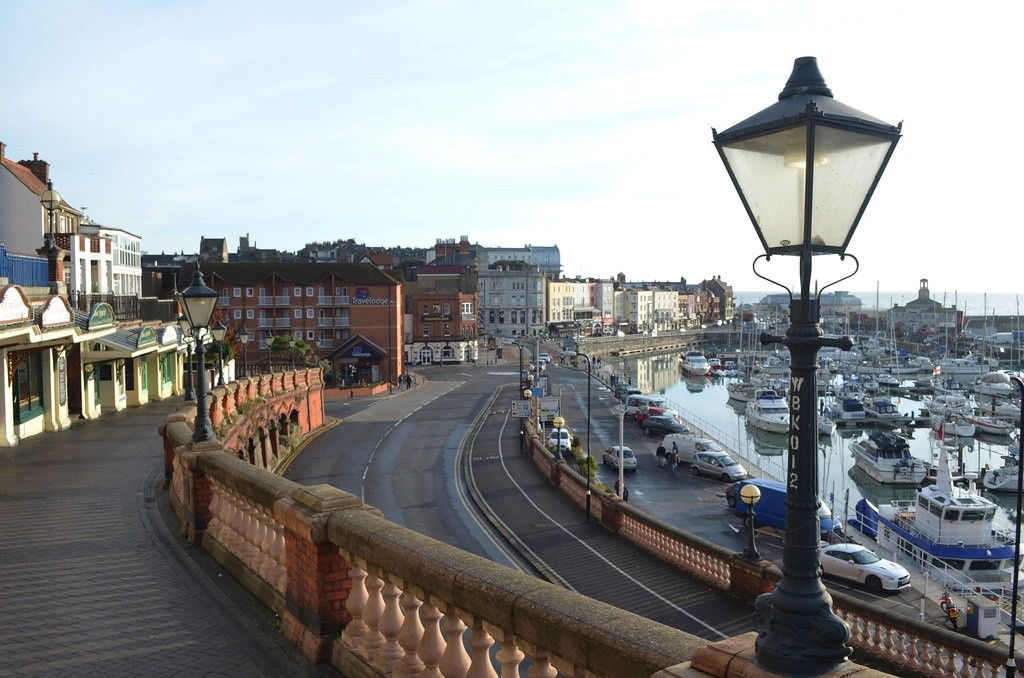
[{"xmin": 683, "ymin": 281, "xmax": 1023, "ymax": 629}]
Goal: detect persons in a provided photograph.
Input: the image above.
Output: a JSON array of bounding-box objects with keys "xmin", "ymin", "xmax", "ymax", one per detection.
[
  {"xmin": 656, "ymin": 443, "xmax": 666, "ymax": 468},
  {"xmin": 592, "ymin": 354, "xmax": 602, "ymax": 366},
  {"xmin": 610, "ymin": 372, "xmax": 616, "ymax": 387},
  {"xmin": 614, "ymin": 478, "xmax": 629, "ymax": 502},
  {"xmin": 672, "ymin": 441, "xmax": 679, "ymax": 466},
  {"xmin": 668, "ymin": 449, "xmax": 680, "ymax": 477},
  {"xmin": 398, "ymin": 375, "xmax": 404, "ymax": 390},
  {"xmin": 933, "ymin": 366, "xmax": 941, "ymax": 376},
  {"xmin": 406, "ymin": 375, "xmax": 413, "ymax": 391}
]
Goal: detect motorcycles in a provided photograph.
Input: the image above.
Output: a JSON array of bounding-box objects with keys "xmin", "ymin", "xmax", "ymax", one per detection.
[{"xmin": 939, "ymin": 591, "xmax": 959, "ymax": 629}]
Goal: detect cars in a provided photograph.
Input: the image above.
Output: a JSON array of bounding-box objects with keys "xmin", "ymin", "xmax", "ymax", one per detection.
[
  {"xmin": 725, "ymin": 483, "xmax": 740, "ymax": 507},
  {"xmin": 690, "ymin": 450, "xmax": 749, "ymax": 482},
  {"xmin": 534, "ymin": 361, "xmax": 546, "ymax": 371},
  {"xmin": 539, "ymin": 352, "xmax": 551, "ymax": 363},
  {"xmin": 820, "ymin": 541, "xmax": 912, "ymax": 593},
  {"xmin": 602, "ymin": 445, "xmax": 638, "ymax": 472},
  {"xmin": 642, "ymin": 416, "xmax": 689, "ymax": 436},
  {"xmin": 549, "ymin": 428, "xmax": 573, "ymax": 451}
]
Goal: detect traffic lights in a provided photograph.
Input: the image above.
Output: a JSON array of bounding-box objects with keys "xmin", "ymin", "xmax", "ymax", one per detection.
[{"xmin": 350, "ymin": 366, "xmax": 357, "ymax": 374}]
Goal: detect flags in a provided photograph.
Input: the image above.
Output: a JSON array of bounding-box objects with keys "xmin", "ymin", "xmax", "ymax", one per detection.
[{"xmin": 937, "ymin": 419, "xmax": 943, "ymax": 441}]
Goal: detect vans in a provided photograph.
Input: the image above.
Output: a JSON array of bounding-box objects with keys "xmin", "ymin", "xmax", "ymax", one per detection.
[
  {"xmin": 736, "ymin": 479, "xmax": 843, "ymax": 539},
  {"xmin": 661, "ymin": 435, "xmax": 725, "ymax": 463},
  {"xmin": 713, "ymin": 320, "xmax": 722, "ymax": 326},
  {"xmin": 619, "ymin": 388, "xmax": 641, "ymax": 403},
  {"xmin": 625, "ymin": 395, "xmax": 683, "ymax": 424}
]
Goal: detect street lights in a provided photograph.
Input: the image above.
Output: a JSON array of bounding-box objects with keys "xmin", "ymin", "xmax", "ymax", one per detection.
[
  {"xmin": 500, "ymin": 339, "xmax": 524, "ymax": 454},
  {"xmin": 441, "ymin": 314, "xmax": 444, "ymax": 368},
  {"xmin": 619, "ymin": 406, "xmax": 650, "ymax": 502},
  {"xmin": 563, "ymin": 351, "xmax": 592, "ymax": 523},
  {"xmin": 981, "ymin": 372, "xmax": 1024, "ymax": 678}
]
